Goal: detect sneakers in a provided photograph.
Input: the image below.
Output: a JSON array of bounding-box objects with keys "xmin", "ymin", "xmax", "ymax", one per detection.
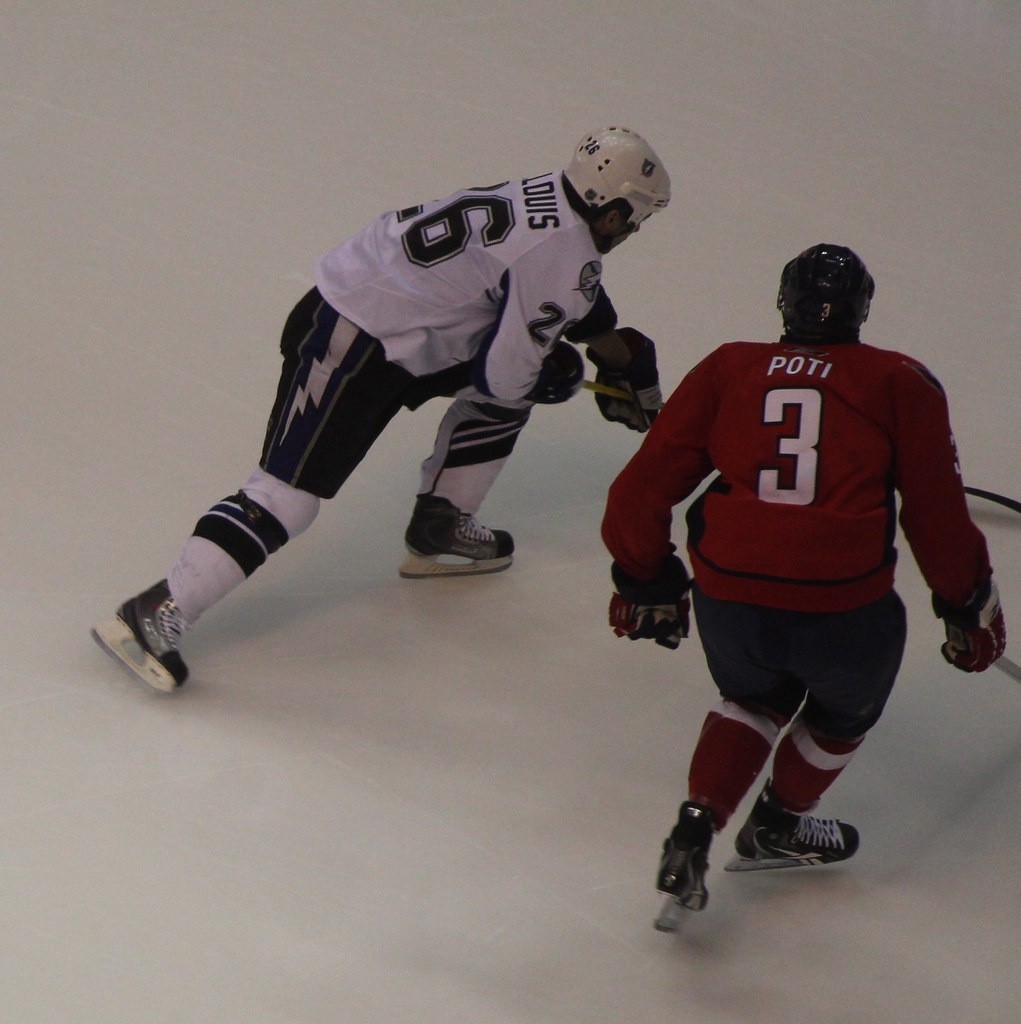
[
  {"xmin": 398, "ymin": 495, "xmax": 517, "ymax": 578},
  {"xmin": 93, "ymin": 577, "xmax": 190, "ymax": 692},
  {"xmin": 721, "ymin": 775, "xmax": 860, "ymax": 873},
  {"xmin": 651, "ymin": 799, "xmax": 709, "ymax": 931}
]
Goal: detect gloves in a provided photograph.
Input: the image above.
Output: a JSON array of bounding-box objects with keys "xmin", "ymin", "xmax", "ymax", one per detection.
[
  {"xmin": 583, "ymin": 327, "xmax": 662, "ymax": 434},
  {"xmin": 607, "ymin": 543, "xmax": 694, "ymax": 650},
  {"xmin": 930, "ymin": 567, "xmax": 1009, "ymax": 671},
  {"xmin": 530, "ymin": 340, "xmax": 587, "ymax": 406}
]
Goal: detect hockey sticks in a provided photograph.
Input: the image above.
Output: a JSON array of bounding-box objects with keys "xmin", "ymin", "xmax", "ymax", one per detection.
[
  {"xmin": 576, "ymin": 376, "xmax": 1021, "ymax": 515},
  {"xmin": 993, "ymin": 653, "xmax": 1021, "ymax": 690}
]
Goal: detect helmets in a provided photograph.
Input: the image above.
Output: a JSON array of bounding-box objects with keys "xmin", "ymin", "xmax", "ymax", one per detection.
[
  {"xmin": 775, "ymin": 243, "xmax": 876, "ymax": 343},
  {"xmin": 564, "ymin": 123, "xmax": 672, "ymax": 232}
]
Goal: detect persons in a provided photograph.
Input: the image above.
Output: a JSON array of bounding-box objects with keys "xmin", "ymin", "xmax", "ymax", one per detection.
[
  {"xmin": 86, "ymin": 125, "xmax": 671, "ymax": 692},
  {"xmin": 601, "ymin": 244, "xmax": 1007, "ymax": 933}
]
